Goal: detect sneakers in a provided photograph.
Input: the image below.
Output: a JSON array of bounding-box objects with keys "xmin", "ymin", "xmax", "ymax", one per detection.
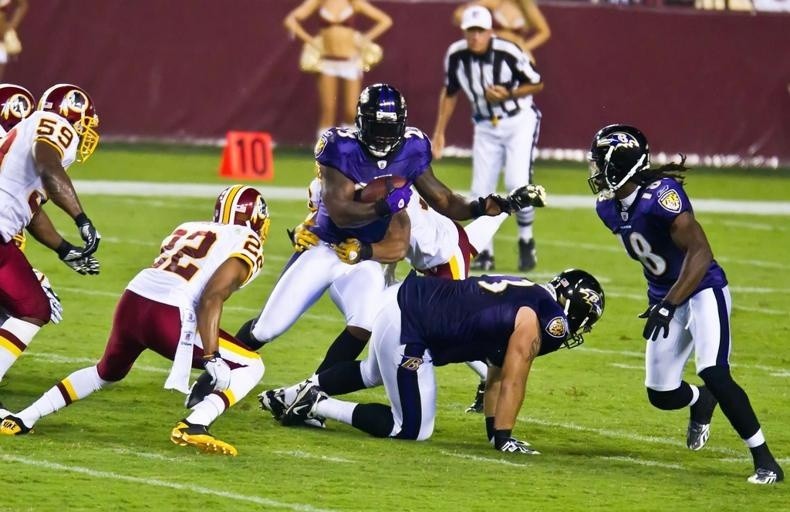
[
  {"xmin": 748, "ymin": 463, "xmax": 784, "ymax": 485},
  {"xmin": 687, "ymin": 386, "xmax": 718, "ymax": 451},
  {"xmin": 470, "ymin": 250, "xmax": 495, "ymax": 271},
  {"xmin": 0, "ymin": 401, "xmax": 35, "ymax": 435},
  {"xmin": 464, "ymin": 381, "xmax": 486, "ymax": 413},
  {"xmin": 170, "ymin": 370, "xmax": 238, "ymax": 457},
  {"xmin": 257, "ymin": 378, "xmax": 329, "ymax": 428},
  {"xmin": 508, "ymin": 184, "xmax": 547, "ymax": 210},
  {"xmin": 518, "ymin": 238, "xmax": 537, "ymax": 271}
]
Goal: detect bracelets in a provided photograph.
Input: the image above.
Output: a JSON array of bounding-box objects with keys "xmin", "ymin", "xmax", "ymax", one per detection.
[
  {"xmin": 203, "ymin": 351, "xmax": 220, "ymax": 361},
  {"xmin": 508, "ymin": 90, "xmax": 515, "ymax": 101},
  {"xmin": 471, "ymin": 200, "xmax": 484, "ymax": 219},
  {"xmin": 376, "ymin": 198, "xmax": 389, "ymax": 217}
]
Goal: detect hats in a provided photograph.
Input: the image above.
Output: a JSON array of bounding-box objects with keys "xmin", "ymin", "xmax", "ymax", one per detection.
[{"xmin": 459, "ymin": 6, "xmax": 493, "ymax": 30}]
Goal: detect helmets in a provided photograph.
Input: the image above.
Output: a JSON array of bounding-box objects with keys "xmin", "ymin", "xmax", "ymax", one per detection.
[
  {"xmin": 587, "ymin": 124, "xmax": 650, "ymax": 203},
  {"xmin": 212, "ymin": 184, "xmax": 270, "ymax": 243},
  {"xmin": 547, "ymin": 267, "xmax": 605, "ymax": 348},
  {"xmin": 0, "ymin": 83, "xmax": 99, "ymax": 164},
  {"xmin": 355, "ymin": 83, "xmax": 407, "ymax": 159}
]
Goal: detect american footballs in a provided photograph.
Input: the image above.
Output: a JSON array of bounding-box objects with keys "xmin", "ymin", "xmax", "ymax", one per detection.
[{"xmin": 360, "ymin": 176, "xmax": 407, "ymax": 203}]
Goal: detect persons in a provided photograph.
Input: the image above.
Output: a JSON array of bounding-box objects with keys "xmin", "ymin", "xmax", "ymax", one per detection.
[
  {"xmin": 0, "ymin": 1, "xmax": 27, "ymax": 67},
  {"xmin": 257, "ymin": 269, "xmax": 606, "ymax": 455},
  {"xmin": 287, "ymin": 177, "xmax": 486, "ymax": 413},
  {"xmin": 587, "ymin": 123, "xmax": 784, "ymax": 486},
  {"xmin": 184, "ymin": 83, "xmax": 547, "ymax": 424},
  {"xmin": 0, "ymin": 84, "xmax": 64, "ymax": 325},
  {"xmin": 0, "ymin": 185, "xmax": 270, "ymax": 457},
  {"xmin": 0, "ymin": 83, "xmax": 101, "ymax": 435},
  {"xmin": 430, "ymin": 5, "xmax": 547, "ymax": 271},
  {"xmin": 452, "ymin": 1, "xmax": 552, "ymax": 65},
  {"xmin": 281, "ymin": 0, "xmax": 393, "ymax": 139}
]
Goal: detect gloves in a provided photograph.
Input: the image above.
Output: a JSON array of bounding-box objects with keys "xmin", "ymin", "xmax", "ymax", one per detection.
[
  {"xmin": 287, "ymin": 221, "xmax": 320, "ymax": 252},
  {"xmin": 486, "ymin": 417, "xmax": 542, "ymax": 455},
  {"xmin": 470, "ymin": 193, "xmax": 512, "ymax": 216},
  {"xmin": 58, "ymin": 219, "xmax": 101, "ymax": 275},
  {"xmin": 33, "ymin": 268, "xmax": 63, "ymax": 324},
  {"xmin": 378, "ymin": 179, "xmax": 413, "ymax": 219},
  {"xmin": 204, "ymin": 357, "xmax": 231, "ymax": 392},
  {"xmin": 332, "ymin": 237, "xmax": 372, "ymax": 265},
  {"xmin": 638, "ymin": 301, "xmax": 675, "ymax": 341}
]
